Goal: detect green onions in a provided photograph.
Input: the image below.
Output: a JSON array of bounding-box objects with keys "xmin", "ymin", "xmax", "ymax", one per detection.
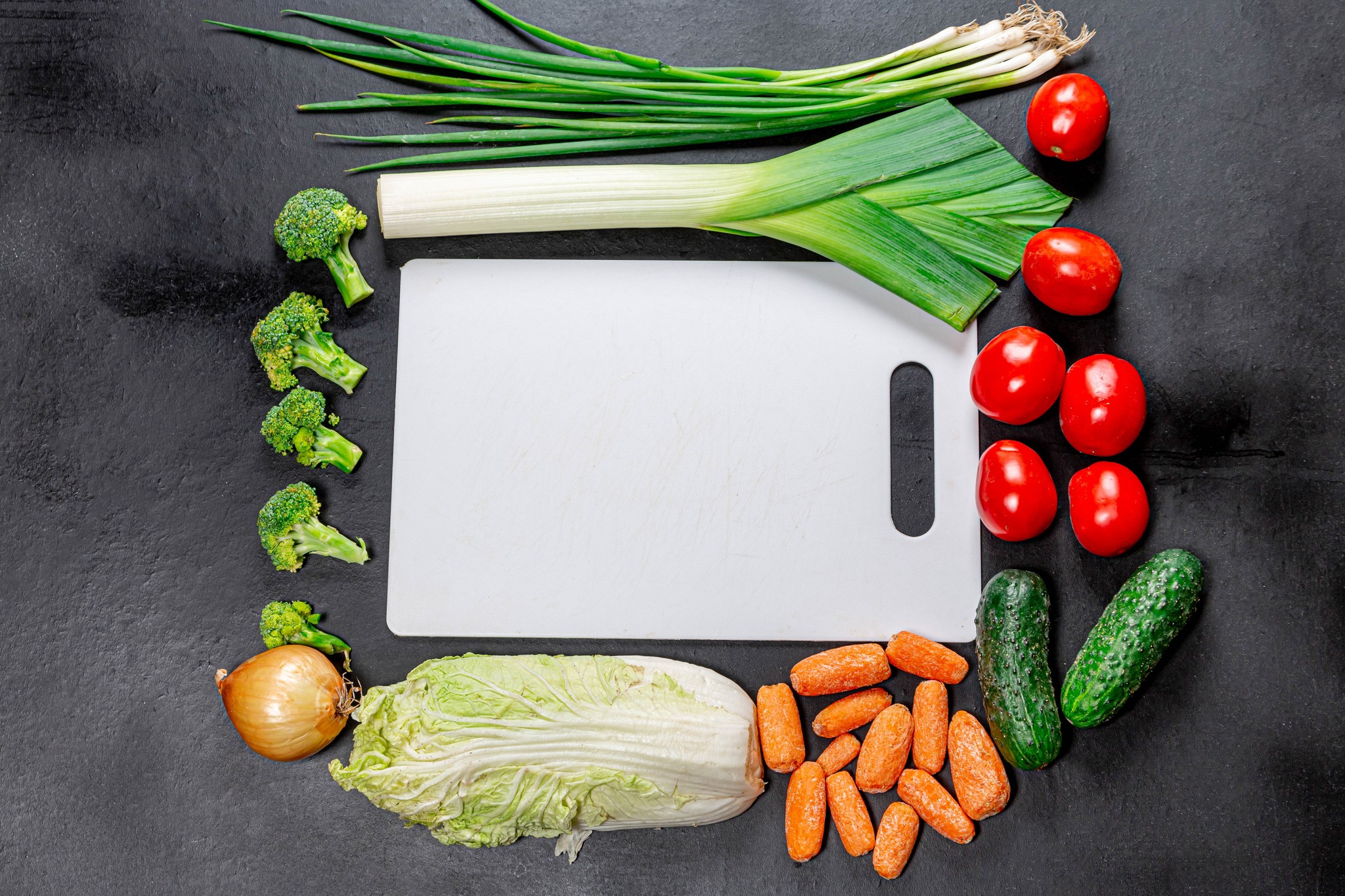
[
  {"xmin": 204, "ymin": 0, "xmax": 1097, "ymax": 173},
  {"xmin": 375, "ymin": 96, "xmax": 1074, "ymax": 332}
]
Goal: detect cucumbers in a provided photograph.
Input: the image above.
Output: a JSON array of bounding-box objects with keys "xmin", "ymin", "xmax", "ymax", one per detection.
[
  {"xmin": 976, "ymin": 570, "xmax": 1062, "ymax": 771},
  {"xmin": 1061, "ymin": 548, "xmax": 1203, "ymax": 726}
]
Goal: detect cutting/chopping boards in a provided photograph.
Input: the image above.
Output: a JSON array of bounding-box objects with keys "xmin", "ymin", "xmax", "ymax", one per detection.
[{"xmin": 376, "ymin": 259, "xmax": 983, "ymax": 644}]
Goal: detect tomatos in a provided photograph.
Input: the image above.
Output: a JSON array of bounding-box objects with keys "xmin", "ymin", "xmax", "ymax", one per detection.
[
  {"xmin": 1025, "ymin": 74, "xmax": 1110, "ymax": 160},
  {"xmin": 975, "ymin": 439, "xmax": 1057, "ymax": 541},
  {"xmin": 1057, "ymin": 353, "xmax": 1147, "ymax": 455},
  {"xmin": 970, "ymin": 326, "xmax": 1067, "ymax": 426},
  {"xmin": 1068, "ymin": 462, "xmax": 1150, "ymax": 555},
  {"xmin": 1020, "ymin": 227, "xmax": 1124, "ymax": 316}
]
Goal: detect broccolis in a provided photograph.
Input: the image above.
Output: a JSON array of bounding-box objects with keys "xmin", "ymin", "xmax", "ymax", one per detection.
[
  {"xmin": 257, "ymin": 599, "xmax": 351, "ymax": 656},
  {"xmin": 250, "ymin": 291, "xmax": 367, "ymax": 394},
  {"xmin": 259, "ymin": 384, "xmax": 364, "ymax": 474},
  {"xmin": 256, "ymin": 481, "xmax": 370, "ymax": 573},
  {"xmin": 275, "ymin": 188, "xmax": 376, "ymax": 307}
]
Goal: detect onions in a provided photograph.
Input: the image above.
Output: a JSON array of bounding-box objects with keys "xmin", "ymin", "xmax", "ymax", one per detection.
[{"xmin": 214, "ymin": 644, "xmax": 363, "ymax": 763}]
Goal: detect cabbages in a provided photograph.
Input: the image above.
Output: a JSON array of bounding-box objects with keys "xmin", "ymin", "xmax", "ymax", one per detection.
[{"xmin": 329, "ymin": 653, "xmax": 765, "ymax": 863}]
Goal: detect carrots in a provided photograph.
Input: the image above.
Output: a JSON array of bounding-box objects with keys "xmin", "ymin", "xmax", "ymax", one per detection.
[{"xmin": 757, "ymin": 629, "xmax": 1010, "ymax": 877}]
